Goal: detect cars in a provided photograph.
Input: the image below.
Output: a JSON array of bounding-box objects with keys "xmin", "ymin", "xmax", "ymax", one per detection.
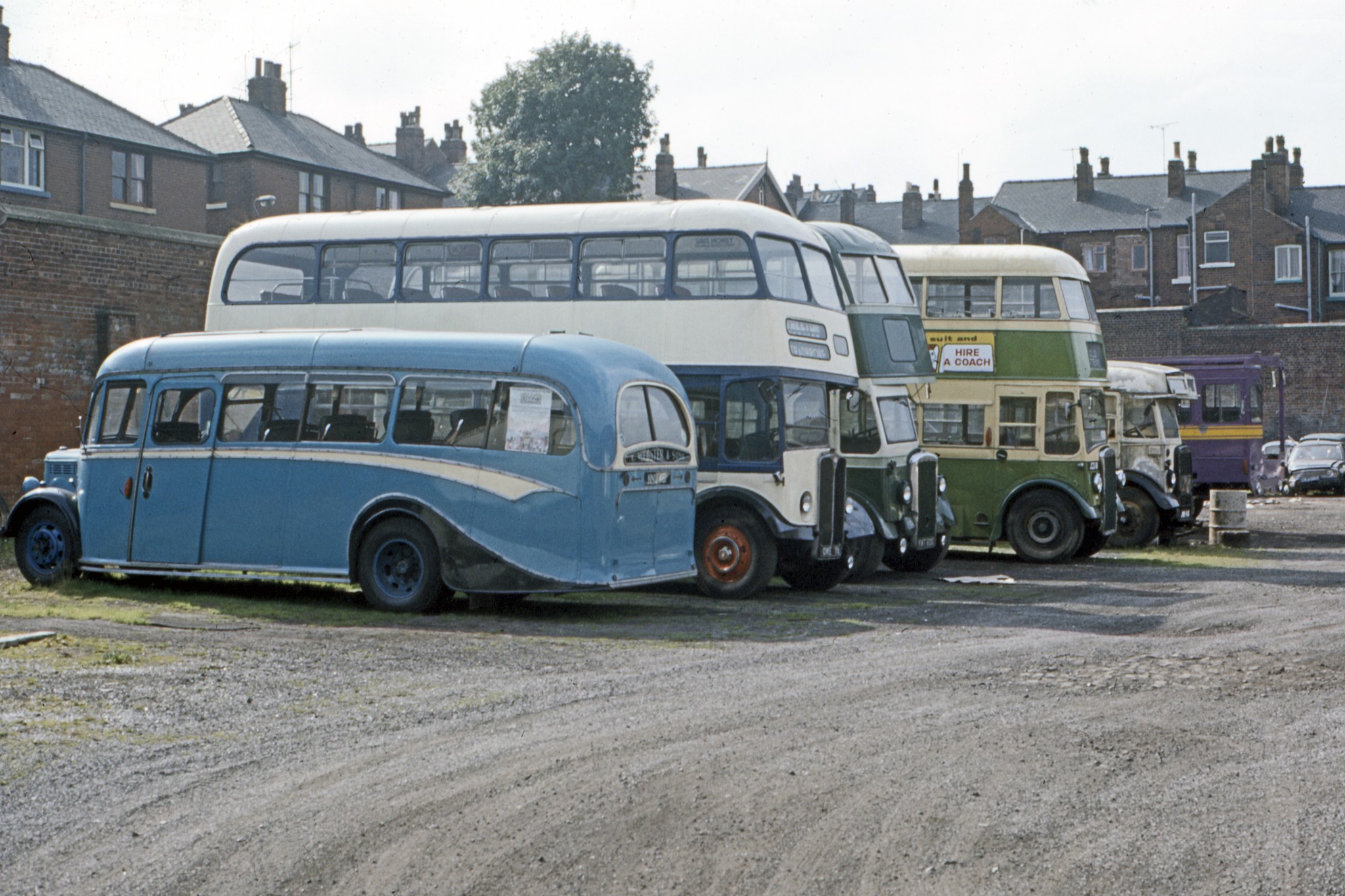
[{"xmin": 1260, "ymin": 433, "xmax": 1345, "ymax": 495}]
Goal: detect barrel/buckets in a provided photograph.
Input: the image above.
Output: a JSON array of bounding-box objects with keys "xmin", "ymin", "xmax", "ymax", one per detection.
[{"xmin": 1209, "ymin": 489, "xmax": 1247, "ymax": 546}]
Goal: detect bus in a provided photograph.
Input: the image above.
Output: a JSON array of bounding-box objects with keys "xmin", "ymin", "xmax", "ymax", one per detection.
[
  {"xmin": 408, "ymin": 246, "xmax": 1284, "ymax": 565},
  {"xmin": 205, "ymin": 202, "xmax": 953, "ymax": 600}
]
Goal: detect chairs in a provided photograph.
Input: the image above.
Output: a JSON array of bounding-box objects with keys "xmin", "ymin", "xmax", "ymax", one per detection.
[
  {"xmin": 260, "ymin": 285, "xmax": 692, "ymax": 303},
  {"xmin": 152, "ymin": 421, "xmax": 201, "ymax": 444},
  {"xmin": 253, "ymin": 407, "xmax": 489, "ymax": 444}
]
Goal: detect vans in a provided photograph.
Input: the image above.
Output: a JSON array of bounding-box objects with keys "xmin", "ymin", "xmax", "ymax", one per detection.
[{"xmin": 1, "ymin": 327, "xmax": 697, "ymax": 614}]
